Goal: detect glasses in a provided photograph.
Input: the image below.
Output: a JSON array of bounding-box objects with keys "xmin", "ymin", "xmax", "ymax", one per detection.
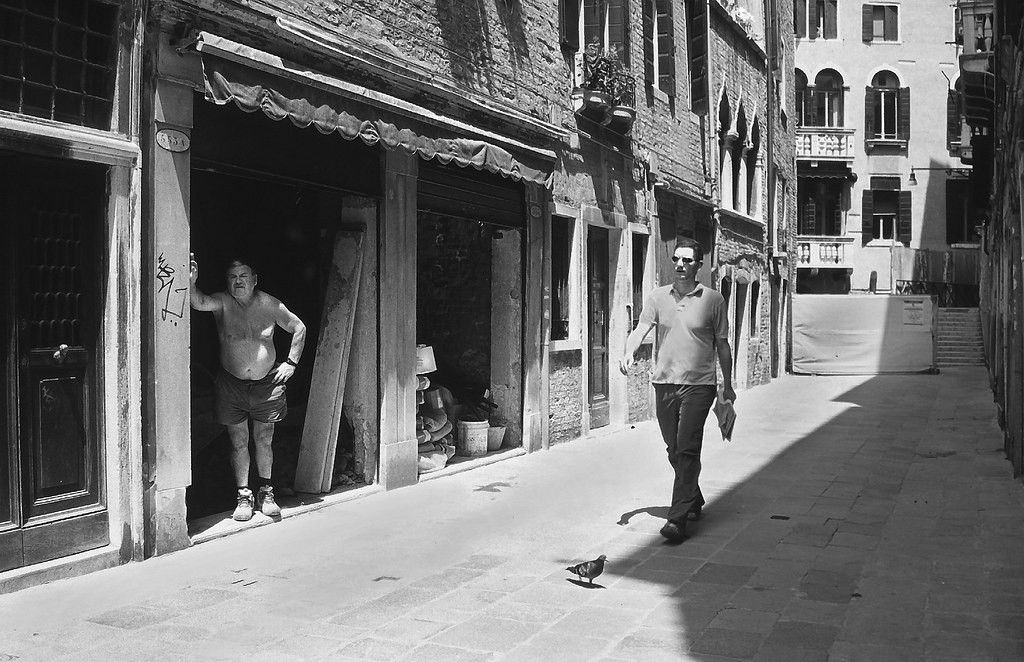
[{"xmin": 672, "ymin": 256, "xmax": 697, "ymax": 263}]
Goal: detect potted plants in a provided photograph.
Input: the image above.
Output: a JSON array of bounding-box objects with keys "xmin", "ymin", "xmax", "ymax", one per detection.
[{"xmin": 581, "ymin": 36, "xmax": 636, "ymax": 123}]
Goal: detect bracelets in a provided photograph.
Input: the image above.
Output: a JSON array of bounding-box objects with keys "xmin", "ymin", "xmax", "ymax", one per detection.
[{"xmin": 286, "ymin": 358, "xmax": 299, "ymax": 368}]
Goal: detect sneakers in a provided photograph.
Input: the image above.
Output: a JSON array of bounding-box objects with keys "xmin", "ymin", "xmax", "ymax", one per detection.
[
  {"xmin": 257, "ymin": 485, "xmax": 280, "ymax": 516},
  {"xmin": 233, "ymin": 488, "xmax": 255, "ymax": 521}
]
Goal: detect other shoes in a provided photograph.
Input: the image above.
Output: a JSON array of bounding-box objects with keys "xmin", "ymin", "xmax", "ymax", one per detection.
[
  {"xmin": 688, "ymin": 506, "xmax": 701, "ymax": 521},
  {"xmin": 660, "ymin": 521, "xmax": 686, "ymax": 540}
]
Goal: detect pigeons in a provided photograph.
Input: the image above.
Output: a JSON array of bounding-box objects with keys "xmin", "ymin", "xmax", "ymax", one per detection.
[{"xmin": 565, "ymin": 554, "xmax": 610, "ymax": 587}]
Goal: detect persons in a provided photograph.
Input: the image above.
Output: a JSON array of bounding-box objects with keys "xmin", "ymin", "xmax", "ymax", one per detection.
[
  {"xmin": 619, "ymin": 238, "xmax": 736, "ymax": 541},
  {"xmin": 189, "ymin": 251, "xmax": 306, "ymax": 520}
]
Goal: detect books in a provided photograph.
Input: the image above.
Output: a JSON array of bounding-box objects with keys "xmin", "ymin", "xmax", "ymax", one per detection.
[{"xmin": 713, "ymin": 395, "xmax": 736, "ymax": 443}]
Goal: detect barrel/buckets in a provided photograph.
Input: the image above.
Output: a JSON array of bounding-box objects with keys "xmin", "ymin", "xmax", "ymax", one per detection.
[
  {"xmin": 457, "ymin": 416, "xmax": 489, "ymax": 457},
  {"xmin": 487, "ymin": 419, "xmax": 508, "ymax": 451},
  {"xmin": 218, "ymin": 463, "xmax": 291, "ymax": 505}
]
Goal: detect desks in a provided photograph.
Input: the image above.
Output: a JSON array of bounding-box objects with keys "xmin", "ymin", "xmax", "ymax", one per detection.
[{"xmin": 897, "ymin": 280, "xmax": 970, "ymax": 307}]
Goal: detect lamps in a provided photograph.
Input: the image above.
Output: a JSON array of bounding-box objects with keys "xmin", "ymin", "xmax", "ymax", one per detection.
[
  {"xmin": 909, "ymin": 165, "xmax": 973, "ymax": 185},
  {"xmin": 811, "ymin": 161, "xmax": 819, "ymax": 167}
]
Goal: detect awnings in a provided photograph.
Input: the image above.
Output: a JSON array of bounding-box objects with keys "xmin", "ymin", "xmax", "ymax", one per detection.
[
  {"xmin": 798, "ymin": 168, "xmax": 852, "ymax": 178},
  {"xmin": 177, "ymin": 32, "xmax": 559, "ymax": 190}
]
